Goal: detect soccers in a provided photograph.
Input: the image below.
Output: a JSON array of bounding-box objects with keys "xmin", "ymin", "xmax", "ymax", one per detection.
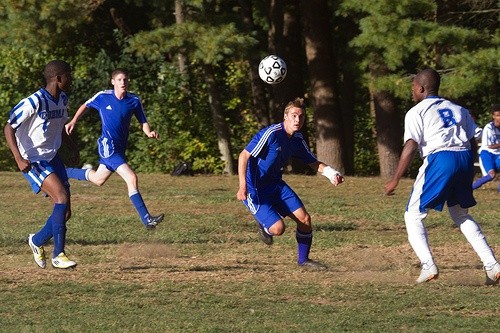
[{"xmin": 259, "ymin": 55, "xmax": 287, "ymax": 85}]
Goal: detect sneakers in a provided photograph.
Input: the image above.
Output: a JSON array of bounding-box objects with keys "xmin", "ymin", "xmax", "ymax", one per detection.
[
  {"xmin": 484, "ymin": 263, "xmax": 500, "ymax": 287},
  {"xmin": 416, "ymin": 264, "xmax": 439, "ymax": 284},
  {"xmin": 146, "ymin": 213, "xmax": 164, "ymax": 230},
  {"xmin": 51, "ymin": 252, "xmax": 77, "ymax": 269},
  {"xmin": 28, "ymin": 234, "xmax": 46, "ymax": 269},
  {"xmin": 259, "ymin": 225, "xmax": 273, "ymax": 245},
  {"xmin": 298, "ymin": 259, "xmax": 328, "ymax": 270}
]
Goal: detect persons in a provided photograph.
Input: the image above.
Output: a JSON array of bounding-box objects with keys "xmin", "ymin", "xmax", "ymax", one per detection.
[
  {"xmin": 473, "ymin": 110, "xmax": 500, "ymax": 191},
  {"xmin": 237, "ymin": 100, "xmax": 345, "ymax": 267},
  {"xmin": 4, "ymin": 59, "xmax": 77, "ymax": 269},
  {"xmin": 65, "ymin": 68, "xmax": 164, "ymax": 229},
  {"xmin": 383, "ymin": 70, "xmax": 500, "ymax": 286}
]
